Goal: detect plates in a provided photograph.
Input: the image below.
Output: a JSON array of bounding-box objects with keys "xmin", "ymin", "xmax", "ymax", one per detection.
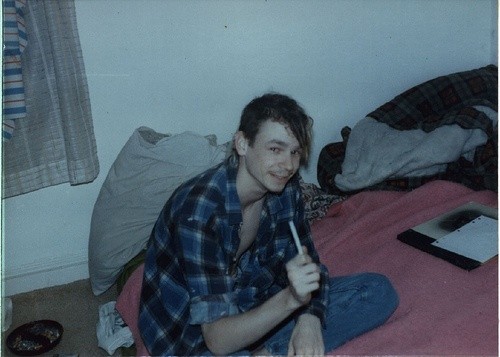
[{"xmin": 7, "ymin": 319, "xmax": 63, "ymax": 355}]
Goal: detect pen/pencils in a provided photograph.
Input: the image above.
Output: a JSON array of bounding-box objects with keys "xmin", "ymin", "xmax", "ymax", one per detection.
[{"xmin": 287, "ymin": 217, "xmax": 306, "ymax": 259}]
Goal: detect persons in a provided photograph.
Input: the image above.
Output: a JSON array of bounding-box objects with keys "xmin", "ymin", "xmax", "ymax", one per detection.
[{"xmin": 139, "ymin": 92, "xmax": 400, "ymax": 356}]
